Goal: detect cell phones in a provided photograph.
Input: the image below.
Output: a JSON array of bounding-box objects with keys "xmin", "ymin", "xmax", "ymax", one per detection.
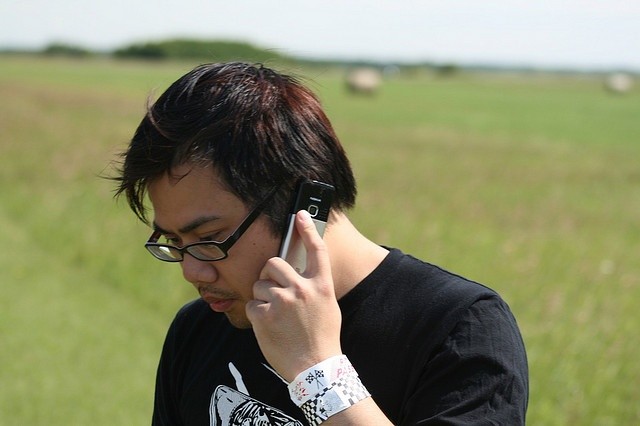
[{"xmin": 278, "ymin": 178, "xmax": 336, "ymax": 273}]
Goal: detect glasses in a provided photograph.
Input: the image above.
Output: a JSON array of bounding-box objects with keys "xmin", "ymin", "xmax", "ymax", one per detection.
[{"xmin": 144, "ymin": 184, "xmax": 282, "ymax": 263}]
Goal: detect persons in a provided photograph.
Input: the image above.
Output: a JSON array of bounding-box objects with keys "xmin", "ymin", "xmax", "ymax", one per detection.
[{"xmin": 116, "ymin": 62, "xmax": 529, "ymax": 426}]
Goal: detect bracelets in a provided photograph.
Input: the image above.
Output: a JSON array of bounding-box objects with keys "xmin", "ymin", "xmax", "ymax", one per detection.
[{"xmin": 286, "ymin": 353, "xmax": 371, "ymax": 425}]
